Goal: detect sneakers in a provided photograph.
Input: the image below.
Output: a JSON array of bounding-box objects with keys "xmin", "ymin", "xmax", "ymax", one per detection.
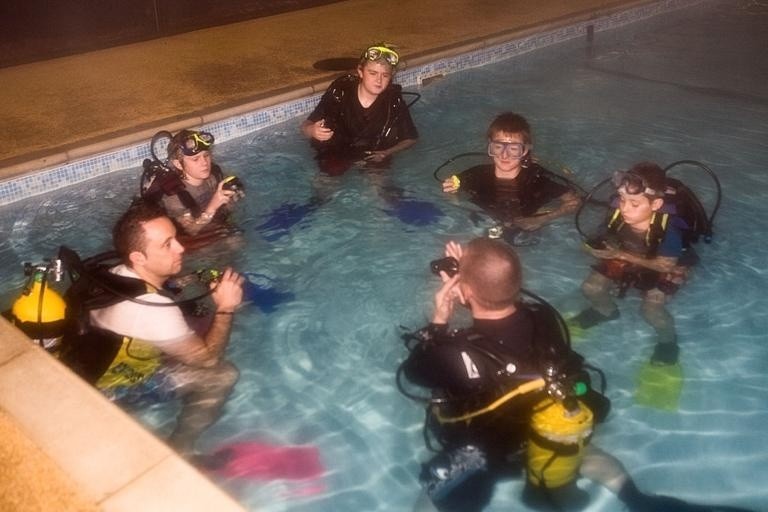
[
  {"xmin": 570, "ymin": 304, "xmax": 619, "ymax": 330},
  {"xmin": 204, "ymin": 452, "xmax": 225, "ymax": 469},
  {"xmin": 651, "ymin": 337, "xmax": 678, "ymax": 364}
]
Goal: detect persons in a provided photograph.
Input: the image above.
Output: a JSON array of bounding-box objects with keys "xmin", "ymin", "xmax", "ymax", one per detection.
[
  {"xmin": 562, "ymin": 158, "xmax": 702, "ymax": 387},
  {"xmin": 63, "ymin": 201, "xmax": 258, "ymax": 479},
  {"xmin": 137, "ymin": 127, "xmax": 297, "ymax": 315},
  {"xmin": 395, "ymin": 234, "xmax": 644, "ymax": 512},
  {"xmin": 433, "ymin": 108, "xmax": 584, "ymax": 247},
  {"xmin": 280, "ymin": 42, "xmax": 423, "ymax": 224}
]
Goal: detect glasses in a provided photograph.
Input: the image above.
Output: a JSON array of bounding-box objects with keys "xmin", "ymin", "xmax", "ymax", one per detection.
[
  {"xmin": 613, "ymin": 171, "xmax": 665, "ymax": 197},
  {"xmin": 487, "ymin": 140, "xmax": 532, "ymax": 158},
  {"xmin": 365, "ymin": 47, "xmax": 399, "ymax": 66},
  {"xmin": 181, "ymin": 133, "xmax": 214, "ymax": 156}
]
